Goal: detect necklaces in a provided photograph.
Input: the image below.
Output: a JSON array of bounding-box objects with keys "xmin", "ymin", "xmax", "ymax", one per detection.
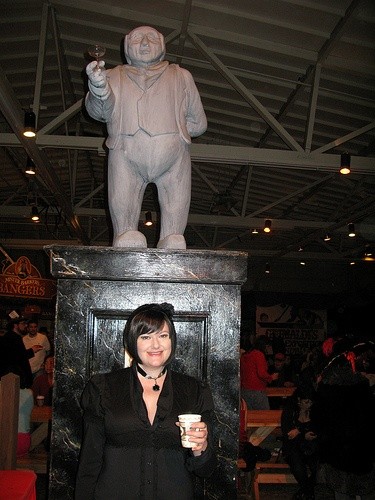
[{"xmin": 136, "ymin": 363, "xmax": 166, "ymax": 391}]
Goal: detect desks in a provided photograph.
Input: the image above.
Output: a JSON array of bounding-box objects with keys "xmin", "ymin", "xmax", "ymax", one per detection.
[{"xmin": 0, "ymin": 364, "xmax": 299, "ymax": 500}]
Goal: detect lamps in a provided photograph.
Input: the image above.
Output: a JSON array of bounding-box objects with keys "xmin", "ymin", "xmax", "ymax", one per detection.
[
  {"xmin": 145, "ymin": 210, "xmax": 152, "ymax": 226},
  {"xmin": 265, "ymin": 261, "xmax": 271, "ymax": 274},
  {"xmin": 23, "ymin": 105, "xmax": 37, "ymax": 138},
  {"xmin": 340, "ymin": 152, "xmax": 351, "ymax": 174},
  {"xmin": 298, "ymin": 223, "xmax": 356, "ymax": 265},
  {"xmin": 264, "ymin": 219, "xmax": 272, "ymax": 233},
  {"xmin": 29, "ymin": 190, "xmax": 39, "ymax": 221},
  {"xmin": 25, "ymin": 156, "xmax": 37, "ymax": 175},
  {"xmin": 252, "ymin": 229, "xmax": 259, "ymax": 234}
]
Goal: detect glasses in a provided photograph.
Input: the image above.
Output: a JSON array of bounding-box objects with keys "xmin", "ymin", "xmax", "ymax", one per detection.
[{"xmin": 13, "ymin": 321, "xmax": 28, "ymax": 325}]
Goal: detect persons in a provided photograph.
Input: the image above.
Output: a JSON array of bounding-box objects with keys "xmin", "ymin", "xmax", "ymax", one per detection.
[
  {"xmin": 75, "ymin": 301, "xmax": 216, "ymax": 500},
  {"xmin": 240, "ymin": 328, "xmax": 375, "ymax": 416},
  {"xmin": 3, "ymin": 365, "xmax": 35, "ymax": 457},
  {"xmin": 30, "ymin": 355, "xmax": 55, "ymax": 446},
  {"xmin": 0, "ymin": 318, "xmax": 54, "ymax": 356},
  {"xmin": 281, "ymin": 384, "xmax": 328, "ymax": 500},
  {"xmin": 21, "ymin": 320, "xmax": 51, "ymax": 377},
  {"xmin": 0, "ymin": 314, "xmax": 44, "ymax": 388},
  {"xmin": 85, "ymin": 24, "xmax": 208, "ymax": 250}
]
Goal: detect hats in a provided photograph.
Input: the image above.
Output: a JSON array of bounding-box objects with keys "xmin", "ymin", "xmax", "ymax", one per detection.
[
  {"xmin": 274, "ymin": 352, "xmax": 285, "ymax": 361},
  {"xmin": 5, "ymin": 308, "xmax": 30, "ymax": 322}
]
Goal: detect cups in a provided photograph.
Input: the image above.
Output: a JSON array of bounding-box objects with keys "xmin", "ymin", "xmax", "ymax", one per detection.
[{"xmin": 178, "ymin": 414, "xmax": 202, "ymax": 449}]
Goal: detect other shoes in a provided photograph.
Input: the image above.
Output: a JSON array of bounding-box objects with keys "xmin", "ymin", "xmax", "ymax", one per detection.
[
  {"xmin": 309, "ymin": 463, "xmax": 318, "ymax": 486},
  {"xmin": 304, "ymin": 488, "xmax": 315, "ymax": 500}
]
